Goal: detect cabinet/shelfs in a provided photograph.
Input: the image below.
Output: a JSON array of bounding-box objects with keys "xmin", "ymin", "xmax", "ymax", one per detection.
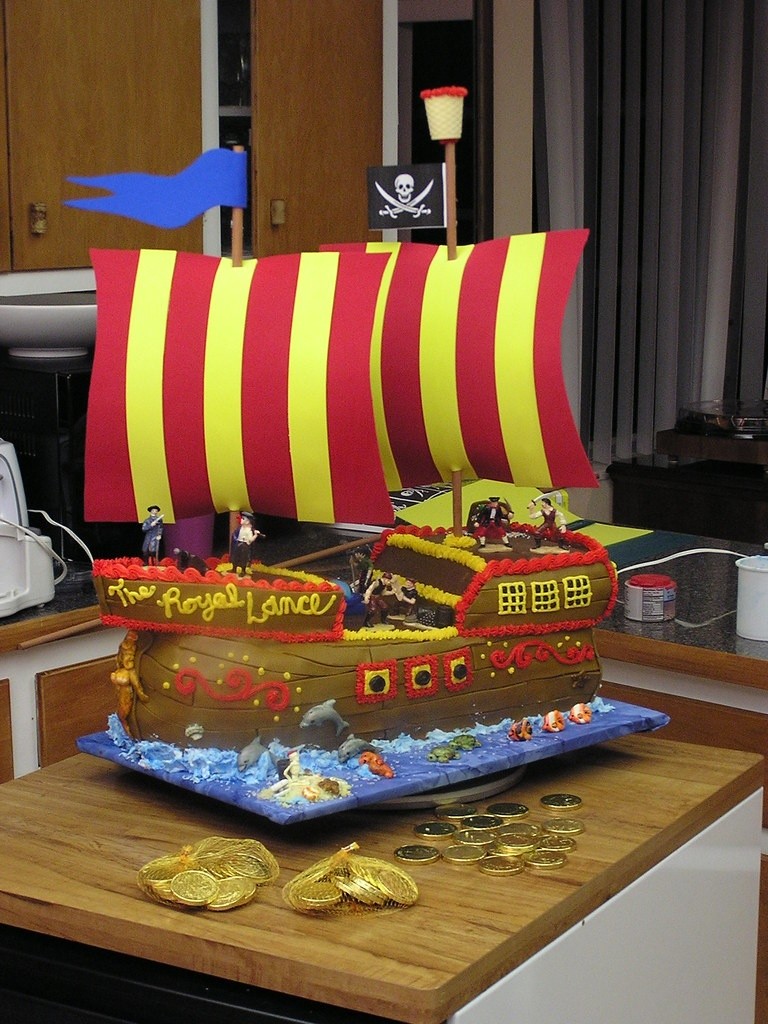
[
  {"xmin": 0, "ymin": 629, "xmax": 768, "ymax": 1024},
  {"xmin": 0, "ymin": 0, "xmax": 387, "ymax": 277},
  {"xmin": 608, "ymin": 475, "xmax": 768, "ymax": 546}
]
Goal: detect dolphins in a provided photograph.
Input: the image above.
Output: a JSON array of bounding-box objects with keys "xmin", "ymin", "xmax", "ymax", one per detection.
[
  {"xmin": 338, "ymin": 733, "xmax": 384, "ymax": 766},
  {"xmin": 237, "ymin": 734, "xmax": 269, "ymax": 773},
  {"xmin": 299, "ymin": 698, "xmax": 350, "ymax": 737}
]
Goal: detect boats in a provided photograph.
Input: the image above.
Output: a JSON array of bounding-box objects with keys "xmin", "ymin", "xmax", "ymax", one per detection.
[{"xmin": 68, "ymin": 87, "xmax": 675, "ymax": 827}]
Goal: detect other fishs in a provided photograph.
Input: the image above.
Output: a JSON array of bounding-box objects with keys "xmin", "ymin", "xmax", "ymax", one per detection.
[
  {"xmin": 506, "ymin": 719, "xmax": 533, "ymax": 741},
  {"xmin": 542, "ymin": 710, "xmax": 566, "ymax": 732},
  {"xmin": 269, "ymin": 751, "xmax": 281, "ymax": 767},
  {"xmin": 569, "ymin": 703, "xmax": 592, "ymax": 724},
  {"xmin": 359, "ymin": 751, "xmax": 395, "ymax": 778}
]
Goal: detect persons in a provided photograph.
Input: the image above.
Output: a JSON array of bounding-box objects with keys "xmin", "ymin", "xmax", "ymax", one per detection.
[
  {"xmin": 141, "ymin": 505, "xmax": 163, "ymax": 566},
  {"xmin": 474, "ymin": 497, "xmax": 514, "ymax": 548},
  {"xmin": 226, "ymin": 512, "xmax": 261, "ymax": 577},
  {"xmin": 530, "ymin": 498, "xmax": 572, "ymax": 550},
  {"xmin": 350, "ymin": 546, "xmax": 418, "ymax": 628}
]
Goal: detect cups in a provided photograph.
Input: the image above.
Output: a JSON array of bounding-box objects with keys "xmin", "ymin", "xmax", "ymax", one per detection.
[{"xmin": 734, "ymin": 555, "xmax": 768, "ymax": 641}]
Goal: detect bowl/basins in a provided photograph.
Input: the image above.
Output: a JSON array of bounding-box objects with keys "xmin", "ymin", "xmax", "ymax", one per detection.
[{"xmin": 0, "ymin": 305, "xmax": 98, "ymax": 358}]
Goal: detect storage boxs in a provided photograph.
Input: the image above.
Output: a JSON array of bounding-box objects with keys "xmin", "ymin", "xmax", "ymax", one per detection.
[{"xmin": 0, "ymin": 605, "xmax": 128, "ymax": 785}]
